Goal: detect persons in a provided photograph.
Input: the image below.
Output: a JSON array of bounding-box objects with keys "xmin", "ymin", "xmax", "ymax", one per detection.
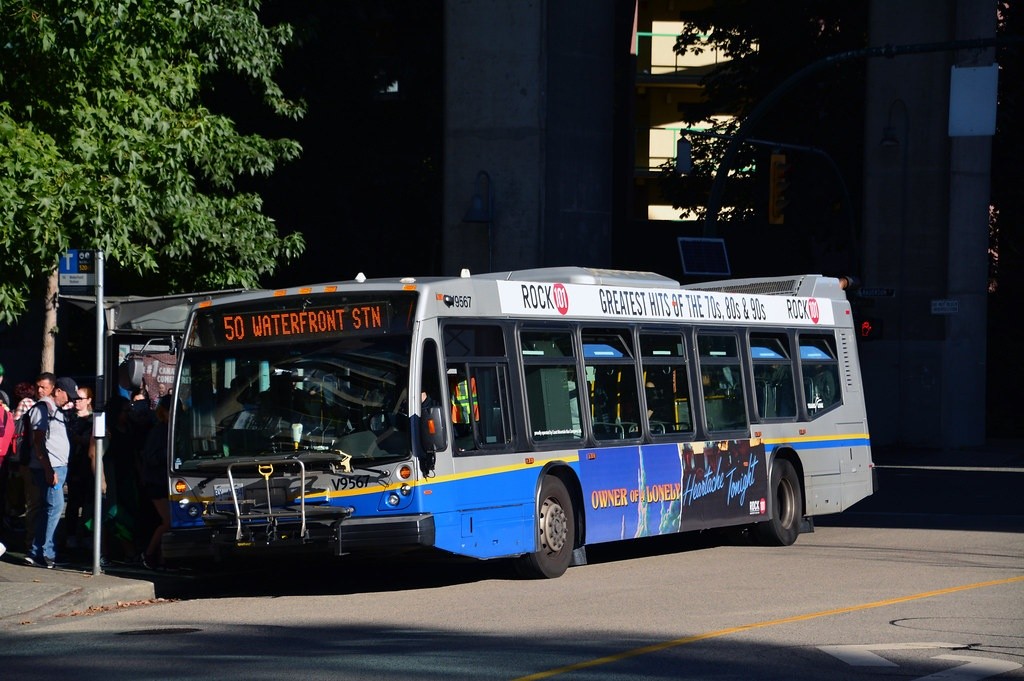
[
  {"xmin": 393, "ymin": 371, "xmax": 458, "ymax": 439},
  {"xmin": 628, "ymin": 363, "xmax": 836, "ymax": 439},
  {"xmin": 1, "ymin": 361, "xmax": 329, "ymax": 570}
]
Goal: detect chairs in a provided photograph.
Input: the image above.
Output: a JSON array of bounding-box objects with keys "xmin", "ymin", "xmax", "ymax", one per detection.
[{"xmin": 416, "ymin": 375, "xmax": 832, "ymax": 444}]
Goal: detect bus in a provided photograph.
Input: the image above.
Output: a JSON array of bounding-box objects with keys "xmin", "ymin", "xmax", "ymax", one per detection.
[{"xmin": 119, "ymin": 270, "xmax": 876, "ymax": 579}]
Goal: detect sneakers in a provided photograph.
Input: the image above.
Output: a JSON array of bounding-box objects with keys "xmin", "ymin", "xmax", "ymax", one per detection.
[
  {"xmin": 140, "ymin": 552, "xmax": 153, "ymax": 568},
  {"xmin": 47, "ymin": 554, "xmax": 70, "ymax": 568},
  {"xmin": 25, "ymin": 554, "xmax": 45, "ymax": 567}
]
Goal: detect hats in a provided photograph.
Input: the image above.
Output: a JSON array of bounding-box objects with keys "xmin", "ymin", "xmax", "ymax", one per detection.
[{"xmin": 55, "ymin": 377, "xmax": 83, "ymax": 400}]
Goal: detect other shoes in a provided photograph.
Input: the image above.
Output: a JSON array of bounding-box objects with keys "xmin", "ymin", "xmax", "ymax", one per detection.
[{"xmin": 100, "ymin": 553, "xmax": 113, "ymax": 566}]
